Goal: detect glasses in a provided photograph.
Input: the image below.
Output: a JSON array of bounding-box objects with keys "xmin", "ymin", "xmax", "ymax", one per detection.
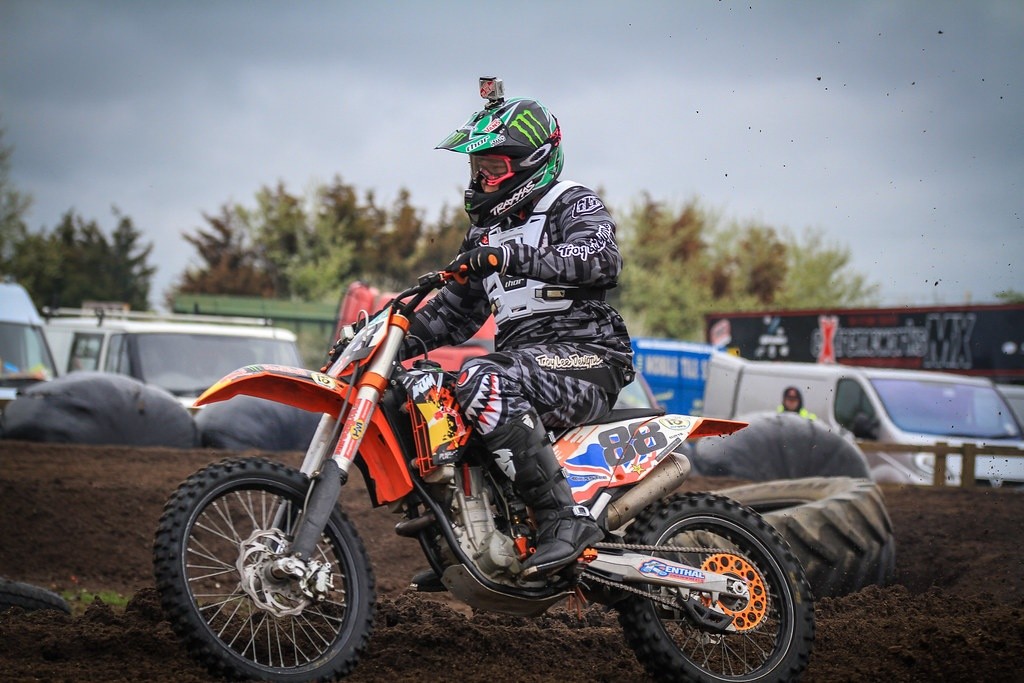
[{"xmin": 469, "ymin": 154, "xmax": 514, "ymax": 186}]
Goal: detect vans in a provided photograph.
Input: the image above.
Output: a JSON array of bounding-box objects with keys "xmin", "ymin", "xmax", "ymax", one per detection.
[
  {"xmin": 0, "ymin": 277, "xmax": 63, "ymax": 400},
  {"xmin": 704, "ymin": 354, "xmax": 1024, "ymax": 488},
  {"xmin": 41, "ymin": 307, "xmax": 300, "ymax": 420}
]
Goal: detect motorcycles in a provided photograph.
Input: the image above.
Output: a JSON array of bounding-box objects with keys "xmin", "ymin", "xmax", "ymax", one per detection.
[{"xmin": 153, "ymin": 253, "xmax": 818, "ymax": 683}]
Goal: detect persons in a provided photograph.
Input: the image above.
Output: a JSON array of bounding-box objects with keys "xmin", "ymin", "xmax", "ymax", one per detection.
[
  {"xmin": 778, "ymin": 387, "xmax": 816, "ymax": 421},
  {"xmin": 319, "ymin": 98, "xmax": 637, "ymax": 592}
]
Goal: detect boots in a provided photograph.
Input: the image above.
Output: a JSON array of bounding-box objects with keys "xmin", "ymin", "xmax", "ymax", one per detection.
[
  {"xmin": 482, "ymin": 406, "xmax": 605, "ymax": 579},
  {"xmin": 409, "ymin": 567, "xmax": 449, "ymax": 593}
]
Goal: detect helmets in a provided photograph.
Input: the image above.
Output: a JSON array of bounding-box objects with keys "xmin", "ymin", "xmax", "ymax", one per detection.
[
  {"xmin": 783, "ymin": 386, "xmax": 803, "ymax": 413},
  {"xmin": 435, "ymin": 98, "xmax": 564, "ymax": 227}
]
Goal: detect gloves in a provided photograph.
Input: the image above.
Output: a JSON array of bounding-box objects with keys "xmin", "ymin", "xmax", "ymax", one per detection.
[{"xmin": 448, "ymin": 247, "xmax": 503, "ymax": 282}]
